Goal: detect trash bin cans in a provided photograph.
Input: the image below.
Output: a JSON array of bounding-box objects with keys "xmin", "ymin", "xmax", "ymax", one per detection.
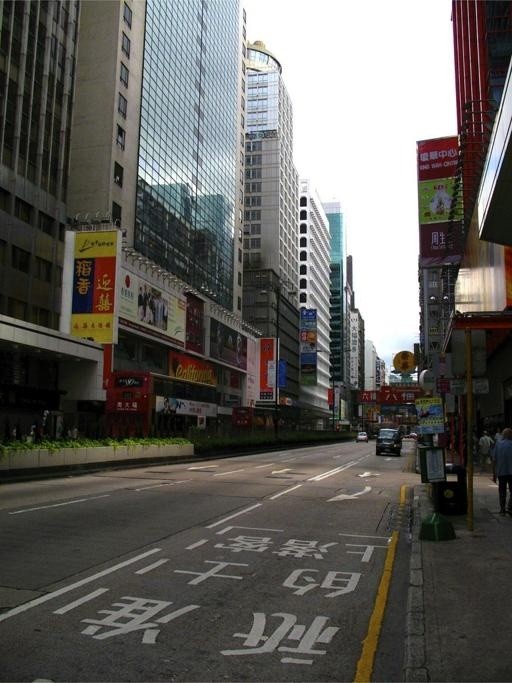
[{"xmin": 432, "ymin": 463, "xmax": 467, "ymax": 516}]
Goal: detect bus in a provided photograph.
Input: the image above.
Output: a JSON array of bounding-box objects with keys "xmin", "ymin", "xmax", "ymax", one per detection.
[
  {"xmin": 229, "ymin": 404, "xmax": 292, "ymax": 433},
  {"xmin": 104, "ymin": 369, "xmax": 219, "ymax": 439}
]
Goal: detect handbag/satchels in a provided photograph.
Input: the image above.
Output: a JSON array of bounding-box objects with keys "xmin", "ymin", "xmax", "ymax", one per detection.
[{"xmin": 420, "ymin": 514, "xmax": 456, "ymax": 542}]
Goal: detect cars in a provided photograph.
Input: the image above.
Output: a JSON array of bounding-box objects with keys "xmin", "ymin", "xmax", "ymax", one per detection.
[
  {"xmin": 403, "ymin": 432, "xmax": 417, "ymax": 438},
  {"xmin": 375, "ymin": 427, "xmax": 402, "ymax": 455},
  {"xmin": 356, "ymin": 431, "xmax": 368, "ymax": 442}
]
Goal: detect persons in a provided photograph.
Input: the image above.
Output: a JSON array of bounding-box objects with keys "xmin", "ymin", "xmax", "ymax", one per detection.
[
  {"xmin": 235, "ymin": 332, "xmax": 244, "ymax": 366},
  {"xmin": 160, "ymin": 397, "xmax": 176, "ymax": 413},
  {"xmin": 216, "ymin": 322, "xmax": 225, "ymax": 360},
  {"xmin": 138, "ymin": 285, "xmax": 167, "ymax": 330},
  {"xmin": 445, "ymin": 424, "xmax": 512, "ymax": 517}
]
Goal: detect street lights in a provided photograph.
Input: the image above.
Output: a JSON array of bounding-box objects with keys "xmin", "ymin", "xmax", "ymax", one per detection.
[{"xmin": 274, "ymin": 280, "xmax": 297, "ymax": 446}]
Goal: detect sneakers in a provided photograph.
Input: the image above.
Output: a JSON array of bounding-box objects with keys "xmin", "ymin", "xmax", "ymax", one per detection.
[{"xmin": 499, "ymin": 508, "xmax": 506, "ymax": 513}]
